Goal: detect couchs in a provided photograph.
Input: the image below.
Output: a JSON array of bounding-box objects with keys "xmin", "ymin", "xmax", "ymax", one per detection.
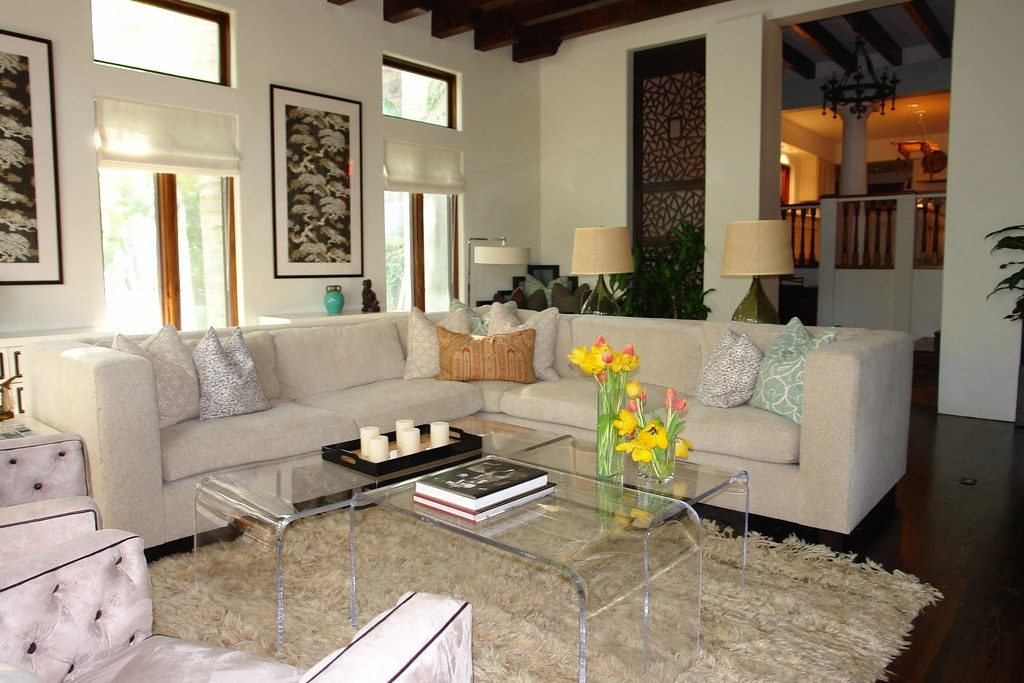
[
  {"xmin": 44, "ymin": 308, "xmax": 483, "ymax": 566},
  {"xmin": 500, "ymin": 315, "xmax": 914, "ymax": 552}
]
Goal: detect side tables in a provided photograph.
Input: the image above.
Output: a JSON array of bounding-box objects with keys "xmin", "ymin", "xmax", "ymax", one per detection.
[{"xmin": 0, "ymin": 416, "xmax": 65, "ymax": 442}]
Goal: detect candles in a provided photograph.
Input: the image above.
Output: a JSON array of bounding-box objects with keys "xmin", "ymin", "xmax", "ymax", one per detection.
[
  {"xmin": 396, "ymin": 419, "xmax": 415, "ymax": 446},
  {"xmin": 368, "ymin": 435, "xmax": 389, "ymax": 463},
  {"xmin": 401, "ymin": 428, "xmax": 420, "ymax": 456},
  {"xmin": 430, "ymin": 421, "xmax": 450, "ymax": 448},
  {"xmin": 359, "ymin": 427, "xmax": 380, "ymax": 456}
]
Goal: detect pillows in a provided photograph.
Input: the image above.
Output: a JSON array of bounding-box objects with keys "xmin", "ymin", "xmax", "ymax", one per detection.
[
  {"xmin": 436, "ymin": 325, "xmax": 538, "ymax": 385},
  {"xmin": 492, "ymin": 287, "xmax": 526, "ymax": 309},
  {"xmin": 696, "ymin": 328, "xmax": 763, "ymax": 410},
  {"xmin": 551, "ymin": 283, "xmax": 590, "ymax": 314},
  {"xmin": 402, "ymin": 304, "xmax": 472, "ymax": 379},
  {"xmin": 190, "ymin": 325, "xmax": 271, "ymax": 420},
  {"xmin": 448, "ymin": 296, "xmax": 519, "ymax": 336},
  {"xmin": 526, "ymin": 289, "xmax": 548, "ymax": 312},
  {"xmin": 746, "ymin": 317, "xmax": 839, "ymax": 425},
  {"xmin": 525, "ymin": 273, "xmax": 569, "ymax": 308},
  {"xmin": 486, "ymin": 302, "xmax": 563, "ymax": 380},
  {"xmin": 111, "ymin": 321, "xmax": 201, "ymax": 430}
]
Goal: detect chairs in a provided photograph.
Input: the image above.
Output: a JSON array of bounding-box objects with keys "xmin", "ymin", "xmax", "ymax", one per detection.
[
  {"xmin": 0, "ymin": 431, "xmax": 104, "ymax": 531},
  {"xmin": 0, "ymin": 527, "xmax": 473, "ymax": 683}
]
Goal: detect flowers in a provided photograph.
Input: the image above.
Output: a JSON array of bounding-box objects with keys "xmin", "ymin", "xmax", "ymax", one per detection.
[
  {"xmin": 565, "ymin": 335, "xmax": 640, "ymax": 476},
  {"xmin": 612, "ymin": 381, "xmax": 694, "ymax": 478}
]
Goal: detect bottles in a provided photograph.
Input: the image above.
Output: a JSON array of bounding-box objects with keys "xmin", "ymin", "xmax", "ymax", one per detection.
[{"xmin": 324, "ymin": 285, "xmax": 344, "ymax": 314}]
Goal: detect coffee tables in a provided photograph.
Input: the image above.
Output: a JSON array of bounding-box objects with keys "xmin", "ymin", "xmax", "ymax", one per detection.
[
  {"xmin": 508, "ymin": 434, "xmax": 751, "ymax": 593},
  {"xmin": 349, "ymin": 434, "xmax": 703, "ymax": 683},
  {"xmin": 192, "ymin": 413, "xmax": 571, "ymax": 656}
]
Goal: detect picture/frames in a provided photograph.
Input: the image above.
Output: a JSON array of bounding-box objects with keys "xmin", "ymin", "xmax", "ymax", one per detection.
[
  {"xmin": 269, "ymin": 83, "xmax": 364, "ymax": 279},
  {"xmin": 0, "ymin": 29, "xmax": 64, "ymax": 287}
]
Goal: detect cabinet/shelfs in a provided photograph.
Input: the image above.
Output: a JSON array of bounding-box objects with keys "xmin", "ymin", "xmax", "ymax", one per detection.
[{"xmin": 257, "ymin": 312, "xmax": 409, "ymax": 326}]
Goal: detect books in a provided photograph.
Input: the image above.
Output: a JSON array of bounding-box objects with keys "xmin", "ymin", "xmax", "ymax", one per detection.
[{"xmin": 412, "ymin": 454, "xmax": 557, "ymax": 522}]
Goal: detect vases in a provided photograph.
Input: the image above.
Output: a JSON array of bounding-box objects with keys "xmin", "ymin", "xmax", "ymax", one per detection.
[
  {"xmin": 637, "ymin": 442, "xmax": 675, "ymax": 484},
  {"xmin": 324, "ymin": 285, "xmax": 345, "ymax": 314},
  {"xmin": 596, "ymin": 389, "xmax": 626, "ymax": 477}
]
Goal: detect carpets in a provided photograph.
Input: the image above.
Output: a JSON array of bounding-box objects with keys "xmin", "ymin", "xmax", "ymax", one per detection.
[{"xmin": 146, "ymin": 486, "xmax": 948, "ymax": 683}]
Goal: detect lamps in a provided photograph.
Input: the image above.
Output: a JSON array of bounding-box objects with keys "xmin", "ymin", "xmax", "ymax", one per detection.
[
  {"xmin": 570, "ymin": 226, "xmax": 634, "ymax": 317},
  {"xmin": 818, "ymin": 36, "xmax": 902, "ymax": 122},
  {"xmin": 890, "ymin": 103, "xmax": 947, "ymax": 189},
  {"xmin": 468, "ymin": 236, "xmax": 532, "ymax": 308},
  {"xmin": 721, "ymin": 220, "xmax": 796, "ymax": 325}
]
